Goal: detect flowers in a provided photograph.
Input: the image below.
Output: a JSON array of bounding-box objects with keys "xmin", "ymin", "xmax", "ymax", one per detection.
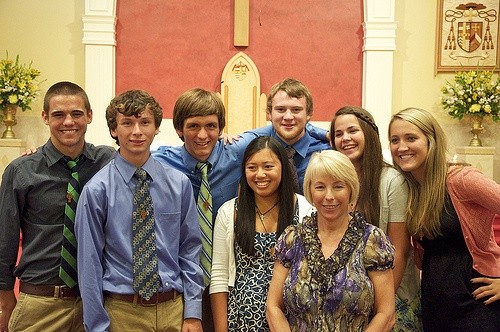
[
  {"xmin": 0, "ymin": 49, "xmax": 47, "ymax": 112},
  {"xmin": 440, "ymin": 59, "xmax": 500, "ymax": 124}
]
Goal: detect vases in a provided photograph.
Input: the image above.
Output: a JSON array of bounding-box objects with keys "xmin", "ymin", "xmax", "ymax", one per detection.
[
  {"xmin": 467, "ymin": 114, "xmax": 484, "ymax": 147},
  {"xmin": 2, "ymin": 103, "xmax": 19, "ymax": 139}
]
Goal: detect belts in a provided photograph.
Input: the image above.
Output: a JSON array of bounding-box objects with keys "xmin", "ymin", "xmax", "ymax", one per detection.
[
  {"xmin": 105, "ymin": 289, "xmax": 182, "ymax": 306},
  {"xmin": 19, "ymin": 280, "xmax": 81, "ymax": 297}
]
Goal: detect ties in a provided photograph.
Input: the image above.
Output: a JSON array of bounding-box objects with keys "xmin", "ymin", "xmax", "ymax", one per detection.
[
  {"xmin": 196, "ymin": 161, "xmax": 213, "ymax": 288},
  {"xmin": 59, "ymin": 156, "xmax": 80, "ymax": 288},
  {"xmin": 132, "ymin": 168, "xmax": 161, "ymax": 301},
  {"xmin": 283, "ymin": 145, "xmax": 300, "ymax": 195}
]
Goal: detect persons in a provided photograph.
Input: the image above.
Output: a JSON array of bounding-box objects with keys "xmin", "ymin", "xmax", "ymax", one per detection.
[
  {"xmin": 0, "ymin": 81, "xmax": 242, "ymax": 332},
  {"xmin": 388, "ymin": 89, "xmax": 500, "ymax": 332},
  {"xmin": 20, "ymin": 78, "xmax": 410, "ymax": 332}
]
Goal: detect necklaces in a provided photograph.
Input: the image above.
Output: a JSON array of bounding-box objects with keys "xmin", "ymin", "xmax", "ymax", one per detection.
[{"xmin": 255, "ymin": 198, "xmax": 280, "ymax": 220}]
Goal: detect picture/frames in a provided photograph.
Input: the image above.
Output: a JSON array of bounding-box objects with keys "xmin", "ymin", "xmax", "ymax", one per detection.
[{"xmin": 434, "ymin": 0, "xmax": 499, "ymax": 74}]
